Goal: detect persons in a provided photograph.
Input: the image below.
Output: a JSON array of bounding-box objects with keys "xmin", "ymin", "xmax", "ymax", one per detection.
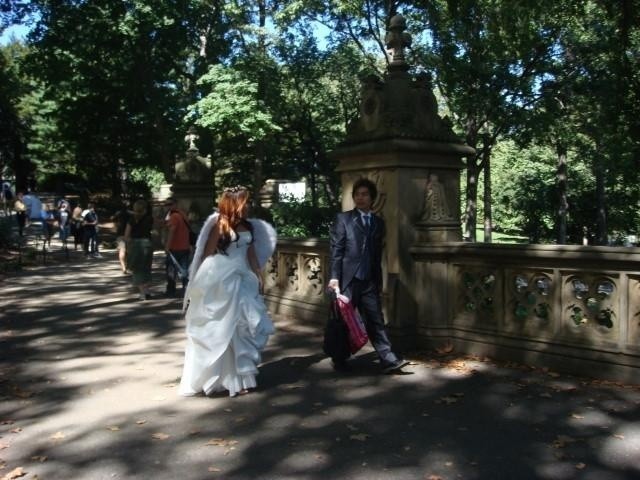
[
  {"xmin": 420, "ymin": 172, "xmax": 458, "ymax": 223},
  {"xmin": 110, "ymin": 198, "xmax": 133, "ymax": 274},
  {"xmin": 161, "ymin": 207, "xmax": 192, "ymax": 297},
  {"xmin": 178, "ymin": 186, "xmax": 276, "ymax": 398},
  {"xmin": 324, "ymin": 178, "xmax": 411, "ymax": 375},
  {"xmin": 123, "ymin": 200, "xmax": 155, "ymax": 300},
  {"xmin": 1, "ymin": 182, "xmax": 103, "ymax": 260}
]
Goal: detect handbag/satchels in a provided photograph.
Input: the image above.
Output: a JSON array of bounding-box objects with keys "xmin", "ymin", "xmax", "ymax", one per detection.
[
  {"xmin": 322, "ymin": 317, "xmax": 350, "ymax": 359},
  {"xmin": 338, "ymin": 297, "xmax": 368, "ymax": 355}
]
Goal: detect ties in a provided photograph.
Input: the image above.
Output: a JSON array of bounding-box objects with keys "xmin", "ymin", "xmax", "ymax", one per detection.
[{"xmin": 362, "ymin": 215, "xmax": 370, "ymax": 231}]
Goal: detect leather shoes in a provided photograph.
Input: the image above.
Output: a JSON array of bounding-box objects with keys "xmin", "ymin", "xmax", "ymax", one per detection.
[{"xmin": 382, "ymin": 358, "xmax": 410, "ymax": 375}]
